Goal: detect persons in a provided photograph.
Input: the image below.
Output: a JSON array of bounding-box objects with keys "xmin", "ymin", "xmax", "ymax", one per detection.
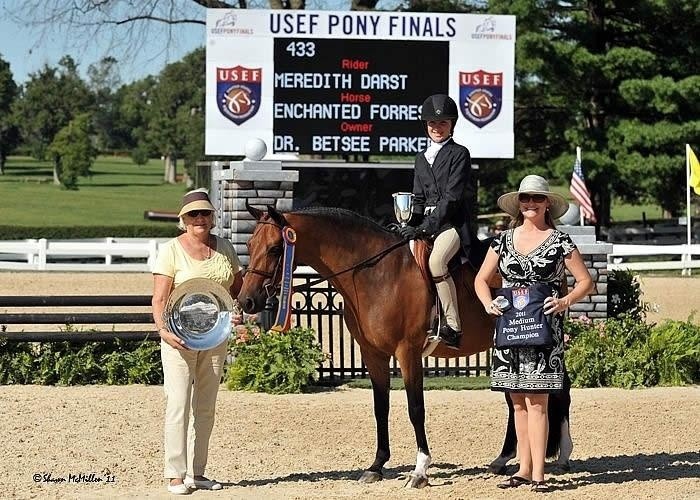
[
  {"xmin": 386, "ymin": 93, "xmax": 480, "ymax": 350},
  {"xmin": 473, "ymin": 175, "xmax": 594, "ymax": 493},
  {"xmin": 147, "ymin": 186, "xmax": 244, "ymax": 496}
]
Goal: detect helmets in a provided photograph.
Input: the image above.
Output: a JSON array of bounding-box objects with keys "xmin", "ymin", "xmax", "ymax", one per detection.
[{"xmin": 422, "ymin": 95, "xmax": 458, "ymax": 122}]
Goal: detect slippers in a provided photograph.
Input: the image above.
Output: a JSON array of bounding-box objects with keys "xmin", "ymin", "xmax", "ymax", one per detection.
[
  {"xmin": 498, "ymin": 475, "xmax": 532, "ymax": 488},
  {"xmin": 533, "ymin": 481, "xmax": 549, "ymax": 492}
]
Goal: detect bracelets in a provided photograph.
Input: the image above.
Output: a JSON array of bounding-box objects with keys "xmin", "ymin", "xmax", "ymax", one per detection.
[{"xmin": 157, "ymin": 326, "xmax": 168, "ymax": 335}]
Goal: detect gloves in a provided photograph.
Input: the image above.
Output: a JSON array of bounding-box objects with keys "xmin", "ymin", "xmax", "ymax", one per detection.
[{"xmin": 397, "ymin": 226, "xmax": 425, "ymax": 241}]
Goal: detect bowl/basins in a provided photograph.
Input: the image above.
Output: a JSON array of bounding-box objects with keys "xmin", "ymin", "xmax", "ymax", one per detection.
[{"xmin": 161, "ymin": 276, "xmax": 241, "ymax": 352}]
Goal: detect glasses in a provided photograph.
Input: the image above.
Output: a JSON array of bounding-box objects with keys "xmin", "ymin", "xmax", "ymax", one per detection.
[
  {"xmin": 518, "ymin": 193, "xmax": 547, "ymax": 203},
  {"xmin": 188, "ymin": 210, "xmax": 212, "ymax": 217}
]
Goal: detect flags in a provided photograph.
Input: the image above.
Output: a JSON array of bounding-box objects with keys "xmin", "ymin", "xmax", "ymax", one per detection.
[
  {"xmin": 570, "ymin": 153, "xmax": 597, "ymax": 223},
  {"xmin": 690, "ymin": 146, "xmax": 700, "ymax": 197}
]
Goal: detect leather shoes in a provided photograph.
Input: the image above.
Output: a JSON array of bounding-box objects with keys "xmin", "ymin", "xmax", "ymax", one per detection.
[
  {"xmin": 167, "ymin": 483, "xmax": 189, "ymax": 495},
  {"xmin": 184, "ymin": 474, "xmax": 222, "ymax": 490},
  {"xmin": 428, "ymin": 324, "xmax": 463, "ymax": 351}
]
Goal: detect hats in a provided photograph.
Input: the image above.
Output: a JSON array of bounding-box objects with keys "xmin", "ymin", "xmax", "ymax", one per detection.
[
  {"xmin": 498, "ymin": 175, "xmax": 568, "ymax": 219},
  {"xmin": 177, "ymin": 193, "xmax": 215, "ymax": 216}
]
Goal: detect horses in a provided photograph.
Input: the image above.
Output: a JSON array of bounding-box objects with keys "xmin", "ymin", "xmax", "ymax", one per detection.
[{"xmin": 235, "ymin": 202, "xmax": 574, "ymax": 489}]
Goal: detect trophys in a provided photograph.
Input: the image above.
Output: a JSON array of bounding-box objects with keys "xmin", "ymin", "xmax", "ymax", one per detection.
[{"xmin": 390, "ymin": 192, "xmax": 416, "ymax": 228}]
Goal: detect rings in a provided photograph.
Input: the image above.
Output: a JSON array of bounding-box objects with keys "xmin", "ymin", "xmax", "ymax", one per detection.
[{"xmin": 490, "ymin": 303, "xmax": 497, "ymax": 308}]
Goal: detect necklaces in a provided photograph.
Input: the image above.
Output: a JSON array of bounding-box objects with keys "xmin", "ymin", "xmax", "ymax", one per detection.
[{"xmin": 197, "ymin": 237, "xmax": 213, "ymax": 260}]
